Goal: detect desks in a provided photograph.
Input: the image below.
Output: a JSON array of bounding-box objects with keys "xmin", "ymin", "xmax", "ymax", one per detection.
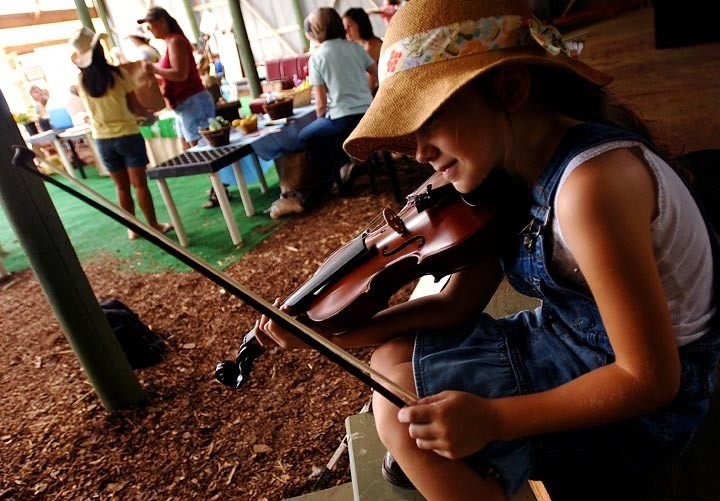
[
  {"xmin": 88, "ymin": 108, "xmax": 190, "ymax": 178},
  {"xmin": 58, "ymin": 128, "xmax": 104, "ymax": 172},
  {"xmin": 27, "ymin": 130, "xmax": 76, "ymax": 178},
  {"xmin": 144, "ymin": 105, "xmax": 317, "ymax": 248}
]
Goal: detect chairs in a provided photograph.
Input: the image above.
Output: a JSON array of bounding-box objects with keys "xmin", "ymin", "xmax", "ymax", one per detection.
[{"xmin": 264, "ymin": 55, "xmax": 309, "ymax": 89}]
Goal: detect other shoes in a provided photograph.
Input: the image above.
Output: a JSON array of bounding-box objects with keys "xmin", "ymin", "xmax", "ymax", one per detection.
[
  {"xmin": 160, "ymin": 223, "xmax": 173, "ymax": 233},
  {"xmin": 204, "ymin": 183, "xmax": 232, "ymax": 208},
  {"xmin": 381, "ymin": 451, "xmax": 415, "ymax": 488},
  {"xmin": 127, "ymin": 231, "xmax": 139, "ymax": 239}
]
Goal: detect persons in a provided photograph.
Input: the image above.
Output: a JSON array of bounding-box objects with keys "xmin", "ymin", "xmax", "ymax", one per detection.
[
  {"xmin": 29, "ymin": 85, "xmax": 73, "ymax": 131},
  {"xmin": 69, "ymin": 26, "xmax": 174, "ymax": 239},
  {"xmin": 137, "ymin": 6, "xmax": 233, "ymax": 209},
  {"xmin": 268, "ymin": 7, "xmax": 377, "ymax": 218},
  {"xmin": 252, "ymin": 0, "xmax": 720, "ymax": 501},
  {"xmin": 342, "ymin": 7, "xmax": 382, "ymax": 98}
]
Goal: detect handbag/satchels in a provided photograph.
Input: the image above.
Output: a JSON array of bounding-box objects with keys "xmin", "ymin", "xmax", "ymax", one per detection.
[{"xmin": 114, "ymin": 62, "xmax": 167, "ymax": 113}]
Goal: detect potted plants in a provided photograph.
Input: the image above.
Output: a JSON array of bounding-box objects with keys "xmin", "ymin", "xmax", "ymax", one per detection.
[
  {"xmin": 13, "ymin": 114, "xmax": 38, "ymax": 136},
  {"xmin": 39, "ymin": 117, "xmax": 50, "ymax": 131}
]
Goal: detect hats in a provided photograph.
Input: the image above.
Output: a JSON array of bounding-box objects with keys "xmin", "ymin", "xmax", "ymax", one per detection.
[
  {"xmin": 136, "ymin": 6, "xmax": 167, "ymax": 24},
  {"xmin": 71, "ymin": 26, "xmax": 109, "ymax": 68},
  {"xmin": 343, "ymin": 0, "xmax": 614, "ymax": 162}
]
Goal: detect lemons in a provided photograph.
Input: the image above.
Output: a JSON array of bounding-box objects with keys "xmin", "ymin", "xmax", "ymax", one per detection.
[{"xmin": 232, "ymin": 116, "xmax": 255, "ymax": 126}]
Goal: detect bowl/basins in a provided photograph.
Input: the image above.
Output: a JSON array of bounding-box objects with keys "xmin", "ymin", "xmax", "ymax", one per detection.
[
  {"xmin": 198, "ymin": 127, "xmax": 230, "ymax": 148},
  {"xmin": 237, "ymin": 115, "xmax": 257, "ymax": 134}
]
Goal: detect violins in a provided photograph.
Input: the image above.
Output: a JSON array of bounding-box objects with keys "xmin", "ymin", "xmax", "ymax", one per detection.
[{"xmin": 213, "ymin": 171, "xmax": 519, "ymax": 392}]
